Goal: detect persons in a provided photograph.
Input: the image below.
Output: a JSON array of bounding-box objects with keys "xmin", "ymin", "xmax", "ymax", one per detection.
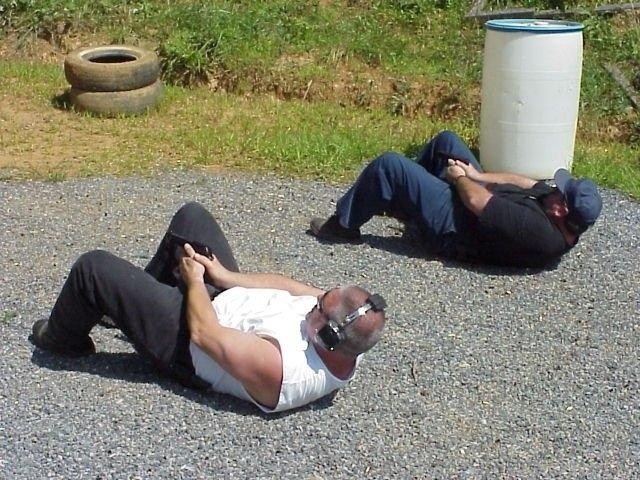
[
  {"xmin": 309, "ymin": 131, "xmax": 602, "ymax": 268},
  {"xmin": 32, "ymin": 201, "xmax": 386, "ymax": 415}
]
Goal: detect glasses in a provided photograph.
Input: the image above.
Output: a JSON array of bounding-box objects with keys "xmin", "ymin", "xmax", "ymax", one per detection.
[{"xmin": 317, "ymin": 294, "xmax": 329, "ymax": 322}]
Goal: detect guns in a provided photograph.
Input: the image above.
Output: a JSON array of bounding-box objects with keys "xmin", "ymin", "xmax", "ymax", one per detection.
[
  {"xmin": 436, "ymin": 149, "xmax": 469, "ymax": 166},
  {"xmin": 169, "ymin": 231, "xmax": 212, "ymax": 259}
]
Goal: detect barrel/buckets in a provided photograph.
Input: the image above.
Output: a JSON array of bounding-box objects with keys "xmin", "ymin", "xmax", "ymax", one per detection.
[{"xmin": 479, "ymin": 18, "xmax": 583, "ymax": 179}]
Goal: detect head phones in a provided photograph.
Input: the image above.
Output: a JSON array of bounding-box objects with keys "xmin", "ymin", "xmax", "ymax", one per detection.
[{"xmin": 315, "ymin": 295, "xmax": 386, "ymax": 351}]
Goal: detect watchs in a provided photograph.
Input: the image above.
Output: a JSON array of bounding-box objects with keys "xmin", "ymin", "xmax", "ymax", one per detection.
[{"xmin": 453, "ymin": 175, "xmax": 466, "ymax": 185}]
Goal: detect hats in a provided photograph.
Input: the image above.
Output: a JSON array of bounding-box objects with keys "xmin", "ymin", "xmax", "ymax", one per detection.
[{"xmin": 554, "ymin": 168, "xmax": 602, "ymax": 226}]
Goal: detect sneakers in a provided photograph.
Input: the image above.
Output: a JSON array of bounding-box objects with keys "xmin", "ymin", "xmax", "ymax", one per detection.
[
  {"xmin": 310, "ymin": 215, "xmax": 360, "ymax": 241},
  {"xmin": 33, "ymin": 320, "xmax": 93, "ymax": 355}
]
